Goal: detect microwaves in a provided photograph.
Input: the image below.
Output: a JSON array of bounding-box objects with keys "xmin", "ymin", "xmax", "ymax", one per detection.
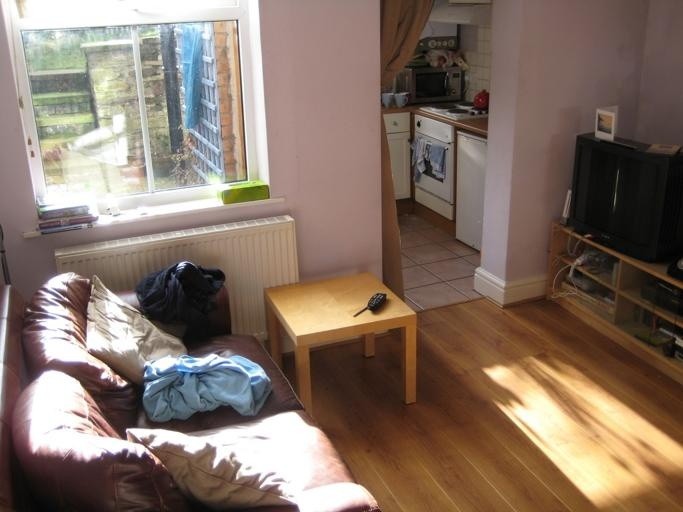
[{"xmin": 395, "ymin": 66, "xmax": 463, "ymax": 104}]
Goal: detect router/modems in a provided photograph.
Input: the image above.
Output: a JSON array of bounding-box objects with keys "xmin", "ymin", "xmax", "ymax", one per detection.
[{"xmin": 560, "ymin": 189, "xmax": 572, "ymax": 226}]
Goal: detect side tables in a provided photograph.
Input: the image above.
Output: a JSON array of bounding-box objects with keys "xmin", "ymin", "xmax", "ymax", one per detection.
[{"xmin": 264, "ymin": 273, "xmax": 418, "ymax": 419}]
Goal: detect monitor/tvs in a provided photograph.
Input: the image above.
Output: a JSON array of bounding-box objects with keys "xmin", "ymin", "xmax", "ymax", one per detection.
[{"xmin": 570, "ymin": 132, "xmax": 683, "ymax": 262}]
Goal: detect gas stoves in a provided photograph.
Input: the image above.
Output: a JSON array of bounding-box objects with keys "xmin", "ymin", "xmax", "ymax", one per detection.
[{"xmin": 418, "ymin": 100, "xmax": 488, "ymax": 121}]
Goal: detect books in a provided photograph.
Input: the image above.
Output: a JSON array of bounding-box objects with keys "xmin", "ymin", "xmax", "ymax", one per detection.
[
  {"xmin": 40, "ymin": 203, "xmax": 90, "ymax": 218},
  {"xmin": 35, "ymin": 214, "xmax": 98, "ymax": 228},
  {"xmin": 633, "ymin": 325, "xmax": 683, "ymax": 362},
  {"xmin": 39, "ymin": 223, "xmax": 95, "ymax": 236}
]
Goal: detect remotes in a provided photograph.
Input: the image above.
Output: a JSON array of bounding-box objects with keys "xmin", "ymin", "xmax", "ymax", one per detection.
[{"xmin": 368, "ymin": 293, "xmax": 386, "ymax": 310}]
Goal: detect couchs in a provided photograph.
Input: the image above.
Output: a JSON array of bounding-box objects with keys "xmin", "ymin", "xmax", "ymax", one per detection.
[{"xmin": 0, "ymin": 285, "xmax": 381, "ymax": 512}]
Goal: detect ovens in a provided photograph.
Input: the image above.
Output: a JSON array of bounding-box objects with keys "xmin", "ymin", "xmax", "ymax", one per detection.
[{"xmin": 413, "ymin": 114, "xmax": 455, "ymax": 221}]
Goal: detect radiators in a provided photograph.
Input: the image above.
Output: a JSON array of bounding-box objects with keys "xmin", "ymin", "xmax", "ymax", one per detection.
[{"xmin": 51, "ymin": 213, "xmax": 300, "ymax": 343}]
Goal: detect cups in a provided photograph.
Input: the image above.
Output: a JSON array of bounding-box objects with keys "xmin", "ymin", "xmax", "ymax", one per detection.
[
  {"xmin": 382, "ymin": 92, "xmax": 395, "ymax": 109},
  {"xmin": 394, "ymin": 92, "xmax": 409, "ymax": 108}
]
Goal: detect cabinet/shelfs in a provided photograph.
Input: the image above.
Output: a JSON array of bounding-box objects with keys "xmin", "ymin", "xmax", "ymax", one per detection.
[
  {"xmin": 382, "ymin": 112, "xmax": 455, "ymax": 222},
  {"xmin": 545, "ymin": 218, "xmax": 682, "ymax": 386}
]
